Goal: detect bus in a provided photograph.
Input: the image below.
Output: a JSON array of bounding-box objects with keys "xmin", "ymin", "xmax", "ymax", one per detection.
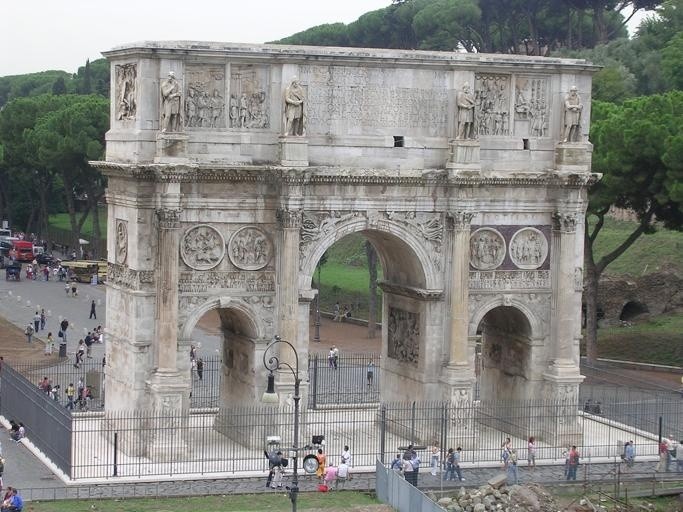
[{"xmin": 59, "ymin": 259, "xmax": 106, "ymax": 283}]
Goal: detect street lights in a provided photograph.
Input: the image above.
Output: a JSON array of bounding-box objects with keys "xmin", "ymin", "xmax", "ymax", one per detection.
[{"xmin": 259, "ymin": 332, "xmax": 324, "ymax": 512}]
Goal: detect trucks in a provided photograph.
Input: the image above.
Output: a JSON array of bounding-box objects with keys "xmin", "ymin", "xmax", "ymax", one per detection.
[{"xmin": 0, "ymin": 227, "xmax": 60, "ymax": 282}]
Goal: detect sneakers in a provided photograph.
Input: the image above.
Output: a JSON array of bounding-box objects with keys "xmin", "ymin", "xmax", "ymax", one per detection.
[
  {"xmin": 271, "ymin": 483, "xmax": 285, "ymax": 489},
  {"xmin": 429, "ymin": 472, "xmax": 466, "ymax": 483}
]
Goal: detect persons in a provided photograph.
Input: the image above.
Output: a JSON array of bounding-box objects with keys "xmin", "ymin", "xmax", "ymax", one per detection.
[
  {"xmin": 501, "ymin": 437, "xmax": 536, "ymax": 485},
  {"xmin": 367, "ymin": 358, "xmax": 375, "ymax": 385},
  {"xmin": 567, "ymin": 445, "xmax": 580, "ymax": 480},
  {"xmin": 0, "ymin": 454, "xmax": 25, "ymax": 512},
  {"xmin": 584, "ymin": 398, "xmax": 591, "ymax": 413},
  {"xmin": 655, "ymin": 435, "xmax": 683, "ymax": 475},
  {"xmin": 190, "ymin": 345, "xmax": 203, "ymax": 381},
  {"xmin": 390, "ymin": 441, "xmax": 465, "ymax": 487},
  {"xmin": 565, "ymin": 86, "xmax": 583, "ymax": 141},
  {"xmin": 13, "ymin": 228, "xmax": 106, "ymax": 412},
  {"xmin": 562, "ymin": 445, "xmax": 571, "ymax": 477},
  {"xmin": 331, "ymin": 302, "xmax": 352, "ymax": 323},
  {"xmin": 159, "ymin": 72, "xmax": 182, "ymax": 131},
  {"xmin": 9, "ymin": 421, "xmax": 19, "ymax": 440},
  {"xmin": 328, "ymin": 347, "xmax": 335, "ymax": 370},
  {"xmin": 594, "ymin": 402, "xmax": 604, "ymax": 414},
  {"xmin": 621, "ymin": 440, "xmax": 635, "ymax": 468},
  {"xmin": 264, "ymin": 446, "xmax": 351, "ymax": 489},
  {"xmin": 14, "ymin": 422, "xmax": 25, "ymax": 443},
  {"xmin": 455, "ymin": 80, "xmax": 476, "ymax": 139},
  {"xmin": 332, "ymin": 345, "xmax": 339, "ymax": 370},
  {"xmin": 283, "ymin": 75, "xmax": 305, "ymax": 135}
]
